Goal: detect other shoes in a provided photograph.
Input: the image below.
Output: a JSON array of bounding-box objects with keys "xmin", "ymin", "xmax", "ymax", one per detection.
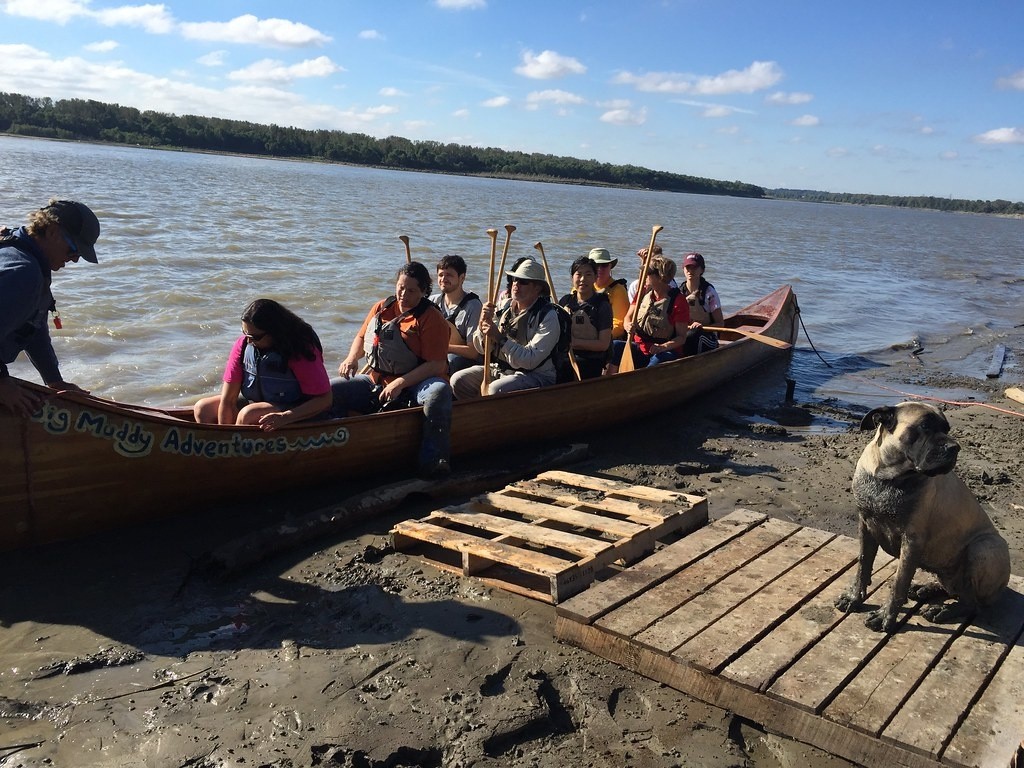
[{"xmin": 418, "ymin": 460, "xmax": 452, "ymax": 482}]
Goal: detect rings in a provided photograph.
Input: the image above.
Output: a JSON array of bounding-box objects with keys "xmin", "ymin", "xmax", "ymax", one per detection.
[{"xmin": 272, "ymin": 421, "xmax": 274, "ymax": 424}]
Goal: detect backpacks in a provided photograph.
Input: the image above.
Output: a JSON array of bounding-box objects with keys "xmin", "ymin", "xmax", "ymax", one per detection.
[{"xmin": 539, "ymin": 303, "xmax": 573, "ymax": 354}]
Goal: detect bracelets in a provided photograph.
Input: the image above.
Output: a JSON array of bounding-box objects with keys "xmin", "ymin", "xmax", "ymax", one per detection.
[{"xmin": 498, "ymin": 337, "xmax": 508, "ymax": 347}]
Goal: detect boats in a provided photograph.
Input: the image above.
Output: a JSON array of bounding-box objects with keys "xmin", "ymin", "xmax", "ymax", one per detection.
[{"xmin": 0, "ymin": 284, "xmax": 801, "ymax": 554}]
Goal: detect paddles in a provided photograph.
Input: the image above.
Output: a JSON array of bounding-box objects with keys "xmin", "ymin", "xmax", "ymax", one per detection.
[
  {"xmin": 399, "ymin": 237, "xmax": 412, "ymax": 260},
  {"xmin": 686, "ymin": 324, "xmax": 792, "ymax": 348},
  {"xmin": 619, "ymin": 224, "xmax": 663, "ymax": 374},
  {"xmin": 535, "ymin": 243, "xmax": 592, "ymax": 382},
  {"xmin": 493, "ymin": 226, "xmax": 518, "ymax": 308},
  {"xmin": 479, "ymin": 229, "xmax": 504, "ymax": 393}
]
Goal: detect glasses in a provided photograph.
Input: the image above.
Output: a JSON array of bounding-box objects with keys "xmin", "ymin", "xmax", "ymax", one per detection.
[
  {"xmin": 58, "ymin": 225, "xmax": 82, "ymax": 259},
  {"xmin": 242, "ymin": 329, "xmax": 268, "ymax": 343},
  {"xmin": 596, "ymin": 262, "xmax": 611, "ymax": 268},
  {"xmin": 512, "ymin": 276, "xmax": 534, "ymax": 285}
]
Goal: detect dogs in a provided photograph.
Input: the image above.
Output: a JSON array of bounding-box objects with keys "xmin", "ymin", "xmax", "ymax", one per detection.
[{"xmin": 833, "ymin": 401, "xmax": 1011, "ymax": 633}]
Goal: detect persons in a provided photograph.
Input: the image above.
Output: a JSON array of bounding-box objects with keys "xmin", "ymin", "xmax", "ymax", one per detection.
[
  {"xmin": 0, "ymin": 200, "xmax": 100, "ymax": 419},
  {"xmin": 194, "ymin": 300, "xmax": 333, "ymax": 432},
  {"xmin": 322, "ymin": 262, "xmax": 452, "ymax": 481},
  {"xmin": 428, "ymin": 245, "xmax": 723, "ymax": 400}
]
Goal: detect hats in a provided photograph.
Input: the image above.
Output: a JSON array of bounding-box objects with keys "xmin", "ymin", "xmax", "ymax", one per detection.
[
  {"xmin": 589, "ymin": 248, "xmax": 618, "ymax": 269},
  {"xmin": 639, "ymin": 264, "xmax": 659, "ymax": 275},
  {"xmin": 40, "ymin": 200, "xmax": 100, "ymax": 263},
  {"xmin": 683, "ymin": 252, "xmax": 704, "ymax": 269},
  {"xmin": 505, "ymin": 259, "xmax": 546, "ymax": 281}
]
